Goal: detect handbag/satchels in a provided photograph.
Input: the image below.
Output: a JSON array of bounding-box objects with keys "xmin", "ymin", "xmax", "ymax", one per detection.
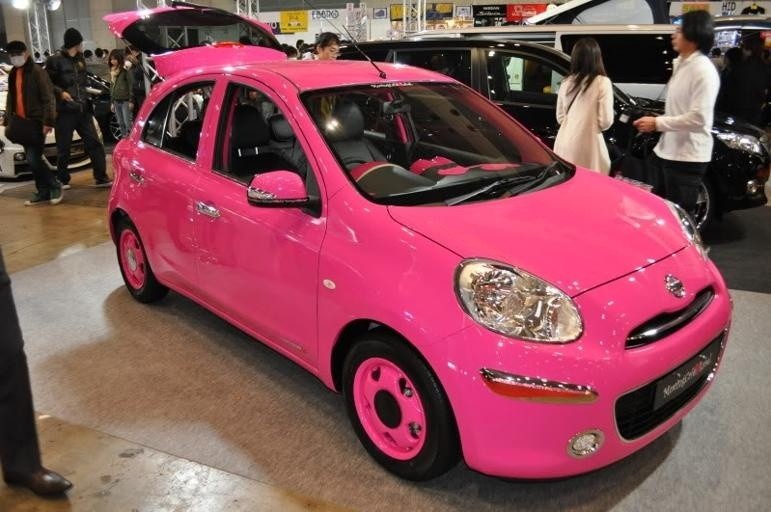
[
  {"xmin": 5, "ymin": 115, "xmax": 41, "ymax": 146},
  {"xmin": 630, "ymin": 131, "xmax": 660, "ymax": 160}
]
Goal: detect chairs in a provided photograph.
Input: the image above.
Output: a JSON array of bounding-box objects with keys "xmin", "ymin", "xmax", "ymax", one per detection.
[
  {"xmin": 231, "ymin": 102, "xmax": 299, "ymax": 179},
  {"xmin": 306, "ymin": 96, "xmax": 390, "ymax": 197},
  {"xmin": 266, "ymin": 114, "xmax": 304, "ymax": 165}
]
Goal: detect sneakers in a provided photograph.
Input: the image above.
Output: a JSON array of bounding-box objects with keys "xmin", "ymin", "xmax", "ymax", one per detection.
[
  {"xmin": 94, "ymin": 179, "xmax": 115, "ymax": 187},
  {"xmin": 23, "ymin": 180, "xmax": 71, "ymax": 206}
]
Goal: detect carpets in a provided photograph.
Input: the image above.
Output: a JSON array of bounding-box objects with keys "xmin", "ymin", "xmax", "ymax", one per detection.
[{"xmin": 4, "ymin": 225, "xmax": 771, "ymax": 510}]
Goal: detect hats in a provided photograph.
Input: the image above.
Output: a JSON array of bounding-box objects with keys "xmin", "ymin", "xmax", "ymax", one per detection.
[
  {"xmin": 64, "ymin": 28, "xmax": 82, "ymax": 49},
  {"xmin": 7, "ymin": 41, "xmax": 24, "ymax": 52}
]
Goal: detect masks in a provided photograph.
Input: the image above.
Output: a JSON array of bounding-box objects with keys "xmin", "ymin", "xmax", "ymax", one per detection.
[{"xmin": 10, "ymin": 55, "xmax": 25, "ymax": 67}]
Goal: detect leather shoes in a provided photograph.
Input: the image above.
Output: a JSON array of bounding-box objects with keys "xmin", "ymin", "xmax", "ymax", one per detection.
[{"xmin": 5, "ymin": 467, "xmax": 72, "ymax": 496}]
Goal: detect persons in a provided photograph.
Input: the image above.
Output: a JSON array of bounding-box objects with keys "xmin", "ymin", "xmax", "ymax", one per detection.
[
  {"xmin": 249, "ymin": 33, "xmax": 340, "ymax": 129},
  {"xmin": 711, "ymin": 26, "xmax": 771, "ymax": 126},
  {"xmin": 631, "ymin": 9, "xmax": 723, "ymax": 215},
  {"xmin": 1, "ymin": 245, "xmax": 72, "ymax": 498},
  {"xmin": 552, "ymin": 37, "xmax": 615, "ymax": 178},
  {"xmin": 4, "ymin": 27, "xmax": 148, "ymax": 206}
]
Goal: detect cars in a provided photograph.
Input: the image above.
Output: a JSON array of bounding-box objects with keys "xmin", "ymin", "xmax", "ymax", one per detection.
[
  {"xmin": 96, "ymin": 1, "xmax": 736, "ymax": 486},
  {"xmin": 0, "ymin": 53, "xmax": 135, "ymax": 180},
  {"xmin": 312, "ymin": 0, "xmax": 771, "ymax": 241}
]
